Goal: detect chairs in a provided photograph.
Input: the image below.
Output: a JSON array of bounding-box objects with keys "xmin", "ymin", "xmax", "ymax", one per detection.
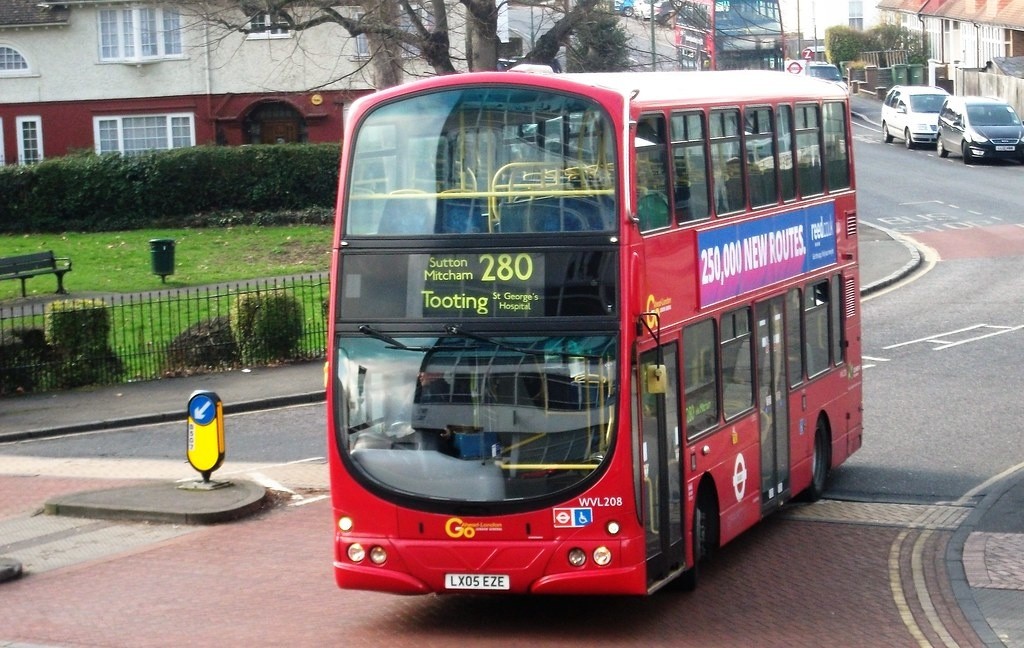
[{"xmin": 381, "ymin": 157, "xmax": 766, "ymax": 229}]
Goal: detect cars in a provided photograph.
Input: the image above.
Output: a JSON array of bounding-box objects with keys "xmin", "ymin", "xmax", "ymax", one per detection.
[{"xmin": 610, "ymin": 0, "xmax": 675, "ymax": 22}]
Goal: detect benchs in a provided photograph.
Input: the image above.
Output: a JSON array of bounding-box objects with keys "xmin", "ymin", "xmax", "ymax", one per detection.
[{"xmin": 0, "ymin": 250, "xmax": 73, "ymax": 297}]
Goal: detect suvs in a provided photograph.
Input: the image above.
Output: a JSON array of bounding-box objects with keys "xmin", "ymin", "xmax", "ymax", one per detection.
[
  {"xmin": 936, "ymin": 96, "xmax": 1024, "ymax": 165},
  {"xmin": 880, "ymin": 84, "xmax": 952, "ymax": 150},
  {"xmin": 764, "ymin": 56, "xmax": 849, "ymax": 92}
]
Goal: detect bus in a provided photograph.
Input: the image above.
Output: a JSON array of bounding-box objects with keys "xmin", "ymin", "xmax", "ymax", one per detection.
[
  {"xmin": 675, "ymin": 0, "xmax": 786, "ymax": 72},
  {"xmin": 323, "ymin": 63, "xmax": 865, "ymax": 597}
]
